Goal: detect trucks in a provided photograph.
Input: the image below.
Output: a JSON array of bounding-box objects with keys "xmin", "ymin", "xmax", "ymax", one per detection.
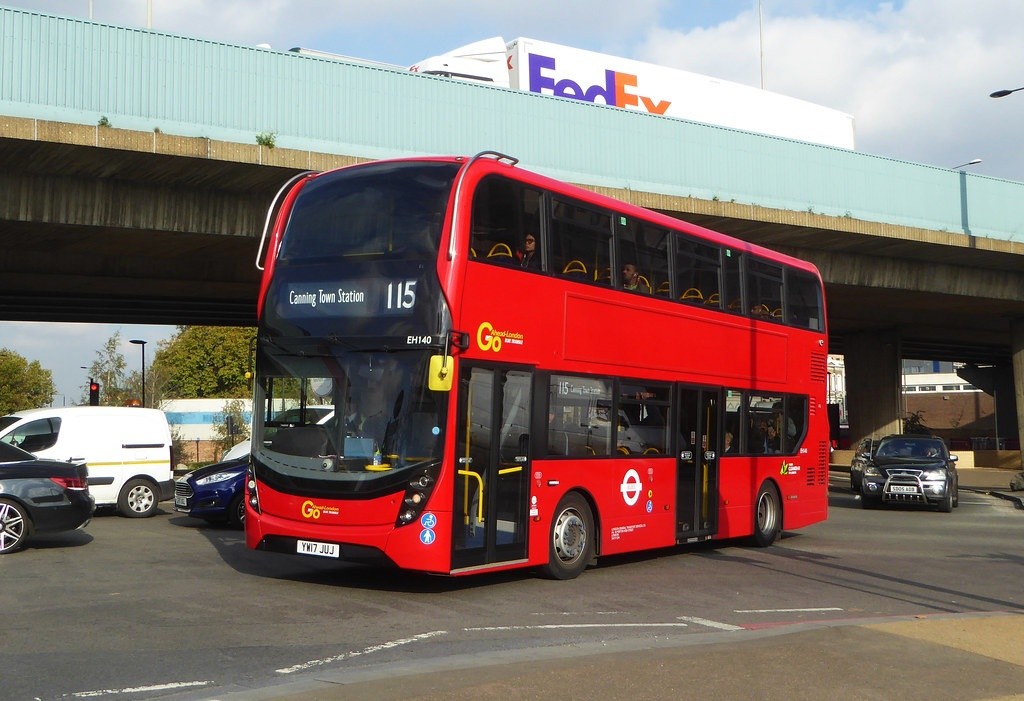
[{"xmin": 405, "ymin": 35, "xmax": 858, "ymax": 153}]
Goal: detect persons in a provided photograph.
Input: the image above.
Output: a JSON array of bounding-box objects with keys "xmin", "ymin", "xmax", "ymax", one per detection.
[
  {"xmin": 625, "ymin": 386, "xmax": 669, "ymax": 460},
  {"xmin": 724, "ymin": 403, "xmax": 797, "ymax": 455},
  {"xmin": 623, "ymin": 262, "xmax": 652, "ymax": 298},
  {"xmin": 519, "ymin": 232, "xmax": 542, "ymax": 271},
  {"xmin": 883, "ymin": 442, "xmax": 902, "ymax": 457},
  {"xmin": 749, "ymin": 301, "xmax": 773, "ymax": 321},
  {"xmin": 332, "ymin": 394, "xmax": 382, "ymax": 471},
  {"xmin": 548, "ymin": 405, "xmax": 567, "ymax": 457},
  {"xmin": 927, "ymin": 441, "xmax": 940, "ymax": 458}
]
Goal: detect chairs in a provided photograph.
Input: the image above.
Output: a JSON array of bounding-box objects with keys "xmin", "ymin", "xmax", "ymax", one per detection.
[
  {"xmin": 274, "ymin": 411, "xmax": 791, "ymax": 460},
  {"xmin": 469, "ymin": 239, "xmax": 797, "ymax": 324}
]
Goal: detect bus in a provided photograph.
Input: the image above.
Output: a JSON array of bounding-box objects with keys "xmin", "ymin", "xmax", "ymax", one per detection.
[{"xmin": 242, "ymin": 149, "xmax": 829, "ymax": 585}]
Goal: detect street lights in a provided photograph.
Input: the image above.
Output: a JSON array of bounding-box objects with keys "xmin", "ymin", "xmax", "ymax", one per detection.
[{"xmin": 130, "ymin": 339, "xmax": 148, "ymax": 406}]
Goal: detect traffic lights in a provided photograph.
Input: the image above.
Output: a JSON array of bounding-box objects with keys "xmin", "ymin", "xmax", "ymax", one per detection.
[{"xmin": 89, "ymin": 381, "xmax": 99, "ymax": 406}]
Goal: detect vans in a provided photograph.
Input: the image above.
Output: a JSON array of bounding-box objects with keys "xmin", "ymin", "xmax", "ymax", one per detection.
[
  {"xmin": 218, "ymin": 403, "xmax": 338, "ymax": 471},
  {"xmin": 0, "ymin": 407, "xmax": 176, "ymax": 516}
]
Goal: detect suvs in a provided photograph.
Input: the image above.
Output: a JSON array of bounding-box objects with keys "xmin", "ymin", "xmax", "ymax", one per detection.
[
  {"xmin": 850, "ymin": 436, "xmax": 883, "ymax": 496},
  {"xmin": 861, "ymin": 432, "xmax": 959, "ymax": 513}
]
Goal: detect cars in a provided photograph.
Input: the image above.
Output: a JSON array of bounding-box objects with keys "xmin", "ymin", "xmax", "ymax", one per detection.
[
  {"xmin": 0, "ymin": 439, "xmax": 96, "ymax": 553},
  {"xmin": 173, "ymin": 452, "xmax": 252, "ymax": 531}
]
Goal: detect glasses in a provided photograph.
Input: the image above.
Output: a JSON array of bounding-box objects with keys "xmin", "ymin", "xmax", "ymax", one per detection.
[{"xmin": 521, "ymin": 238, "xmax": 536, "ymax": 245}]
